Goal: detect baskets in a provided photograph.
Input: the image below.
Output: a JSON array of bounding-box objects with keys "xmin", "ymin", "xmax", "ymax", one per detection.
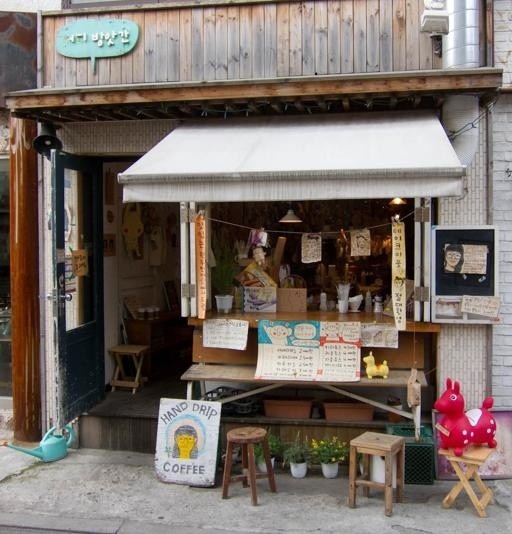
[{"xmin": 203, "ymin": 385, "xmax": 262, "ymax": 417}]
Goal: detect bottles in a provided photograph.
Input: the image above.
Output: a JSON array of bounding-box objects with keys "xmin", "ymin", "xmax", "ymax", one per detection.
[
  {"xmin": 374, "ymin": 296, "xmax": 384, "ymax": 312},
  {"xmin": 365, "ymin": 289, "xmax": 372, "ymax": 312}
]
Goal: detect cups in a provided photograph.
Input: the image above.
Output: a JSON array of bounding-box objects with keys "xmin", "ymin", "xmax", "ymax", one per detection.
[
  {"xmin": 338, "ymin": 300, "xmax": 348, "ymax": 314},
  {"xmin": 234, "ymin": 254, "xmax": 248, "ymax": 263}
]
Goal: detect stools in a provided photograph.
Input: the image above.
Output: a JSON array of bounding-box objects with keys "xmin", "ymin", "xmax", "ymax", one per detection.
[
  {"xmin": 348, "ymin": 431, "xmax": 406, "ymax": 517},
  {"xmin": 438, "ymin": 445, "xmax": 497, "ymax": 518},
  {"xmin": 222, "ymin": 426, "xmax": 276, "ymax": 505},
  {"xmin": 108, "ymin": 344, "xmax": 150, "ymax": 394}
]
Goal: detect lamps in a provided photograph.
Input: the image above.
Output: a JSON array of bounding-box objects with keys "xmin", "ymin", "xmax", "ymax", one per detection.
[{"xmin": 278, "ymin": 201, "xmax": 303, "ymax": 223}]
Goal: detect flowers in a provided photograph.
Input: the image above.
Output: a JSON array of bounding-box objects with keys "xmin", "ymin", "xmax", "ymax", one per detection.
[{"xmin": 311, "ymin": 435, "xmax": 350, "ymax": 464}]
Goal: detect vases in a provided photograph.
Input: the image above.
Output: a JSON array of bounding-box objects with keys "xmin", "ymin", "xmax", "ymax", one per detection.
[
  {"xmin": 321, "ymin": 461, "xmax": 338, "ymax": 478},
  {"xmin": 262, "ymin": 393, "xmax": 403, "ymax": 423}
]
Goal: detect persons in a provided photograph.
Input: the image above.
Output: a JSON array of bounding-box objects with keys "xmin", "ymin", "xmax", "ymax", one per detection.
[
  {"xmin": 443, "ymin": 243, "xmax": 464, "ymax": 273},
  {"xmin": 171, "ymin": 423, "xmax": 200, "ymax": 460}
]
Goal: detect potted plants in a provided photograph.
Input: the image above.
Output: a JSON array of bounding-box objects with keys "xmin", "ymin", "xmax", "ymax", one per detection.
[
  {"xmin": 255, "ymin": 427, "xmax": 313, "ymax": 478},
  {"xmin": 212, "ymin": 243, "xmax": 241, "ymax": 314}
]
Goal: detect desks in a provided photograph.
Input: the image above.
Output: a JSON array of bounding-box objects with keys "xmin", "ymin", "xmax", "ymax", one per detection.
[{"xmin": 179, "ymin": 364, "xmax": 428, "ymax": 442}]
